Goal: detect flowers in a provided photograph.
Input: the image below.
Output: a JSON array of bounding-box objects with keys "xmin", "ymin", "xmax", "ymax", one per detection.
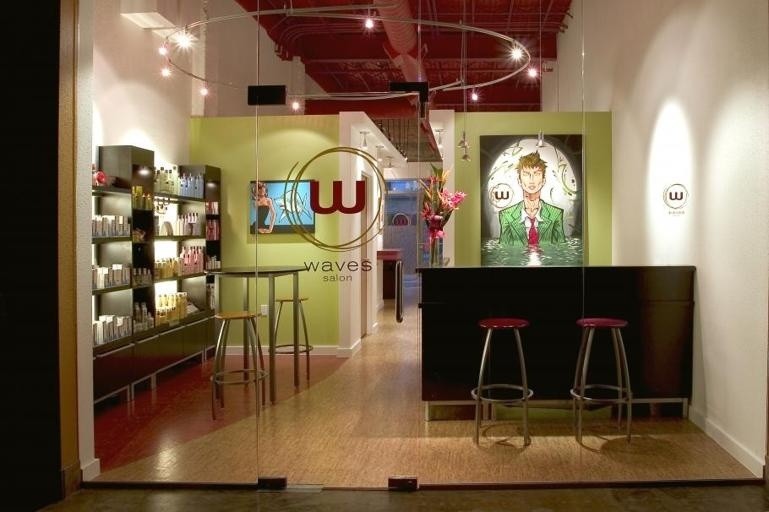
[{"xmin": 418, "ymin": 160, "xmax": 468, "ymax": 236}]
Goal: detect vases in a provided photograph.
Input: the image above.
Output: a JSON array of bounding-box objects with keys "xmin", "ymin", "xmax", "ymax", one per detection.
[{"xmin": 428, "ymin": 230, "xmax": 445, "ymax": 268}]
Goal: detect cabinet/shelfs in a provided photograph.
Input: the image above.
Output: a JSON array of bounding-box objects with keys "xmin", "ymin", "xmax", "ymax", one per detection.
[{"xmin": 92, "ymin": 144, "xmax": 222, "ymax": 329}]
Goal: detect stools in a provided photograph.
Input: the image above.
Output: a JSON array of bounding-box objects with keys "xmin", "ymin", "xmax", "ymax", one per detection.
[
  {"xmin": 569, "ymin": 316, "xmax": 636, "ymax": 446},
  {"xmin": 471, "ymin": 318, "xmax": 535, "ymax": 448},
  {"xmin": 268, "ymin": 295, "xmax": 313, "ymax": 382},
  {"xmin": 208, "ymin": 309, "xmax": 272, "ymax": 421}
]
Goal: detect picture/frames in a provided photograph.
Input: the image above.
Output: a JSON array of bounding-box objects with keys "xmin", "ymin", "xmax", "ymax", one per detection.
[
  {"xmin": 479, "ymin": 132, "xmax": 586, "ymax": 268},
  {"xmin": 247, "ymin": 179, "xmax": 316, "ymax": 238}
]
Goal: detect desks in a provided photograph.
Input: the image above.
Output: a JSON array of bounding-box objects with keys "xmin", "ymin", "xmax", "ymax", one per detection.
[
  {"xmin": 202, "ymin": 264, "xmax": 309, "ymax": 388},
  {"xmin": 376, "ymin": 249, "xmax": 404, "ymax": 325}
]
